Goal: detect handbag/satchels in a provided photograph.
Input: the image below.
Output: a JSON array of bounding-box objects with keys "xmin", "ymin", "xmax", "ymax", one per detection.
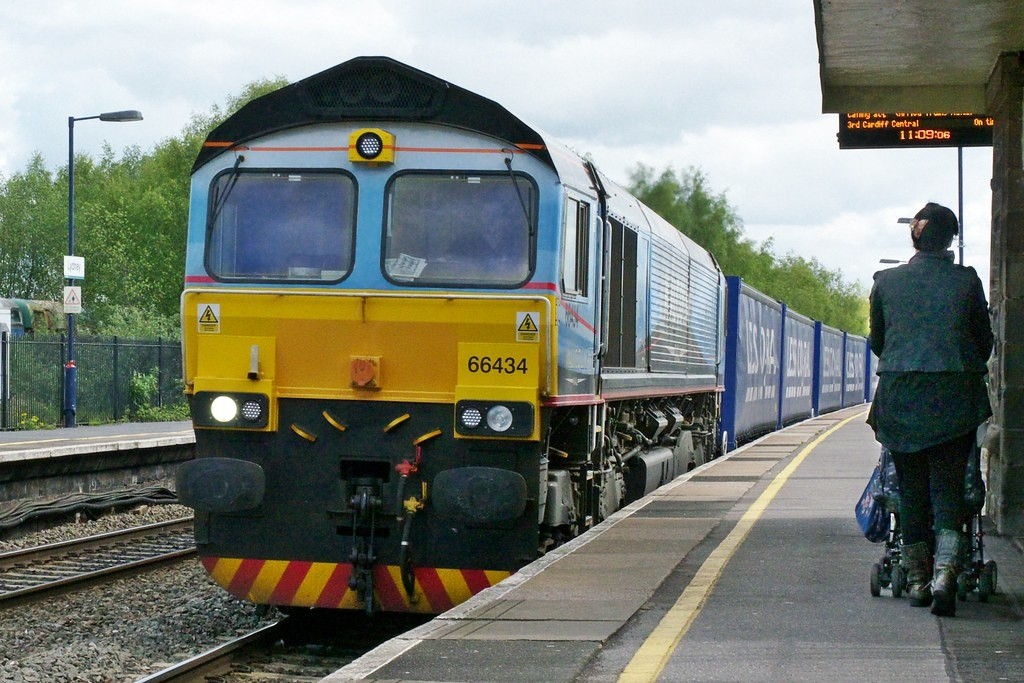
[{"xmin": 855, "ymin": 452, "xmax": 891, "ymax": 543}]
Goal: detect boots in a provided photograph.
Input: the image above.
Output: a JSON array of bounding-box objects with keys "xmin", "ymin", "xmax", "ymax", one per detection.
[
  {"xmin": 930, "ymin": 528, "xmax": 968, "ymax": 616},
  {"xmin": 900, "ymin": 542, "xmax": 932, "ymax": 606}
]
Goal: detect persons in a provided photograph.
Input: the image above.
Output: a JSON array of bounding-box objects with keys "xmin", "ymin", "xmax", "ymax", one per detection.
[{"xmin": 869, "ymin": 202, "xmax": 994, "ymax": 615}]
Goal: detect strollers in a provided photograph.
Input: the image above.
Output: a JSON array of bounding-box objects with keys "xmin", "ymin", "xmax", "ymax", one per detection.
[{"xmin": 869, "ymin": 418, "xmax": 998, "ymax": 605}]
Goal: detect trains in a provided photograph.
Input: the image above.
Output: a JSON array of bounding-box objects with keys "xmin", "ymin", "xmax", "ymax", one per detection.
[{"xmin": 170, "ymin": 53, "xmax": 876, "ymax": 631}]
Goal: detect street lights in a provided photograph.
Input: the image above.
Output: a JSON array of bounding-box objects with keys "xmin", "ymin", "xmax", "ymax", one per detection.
[{"xmin": 59, "ymin": 109, "xmax": 143, "ymax": 429}]
